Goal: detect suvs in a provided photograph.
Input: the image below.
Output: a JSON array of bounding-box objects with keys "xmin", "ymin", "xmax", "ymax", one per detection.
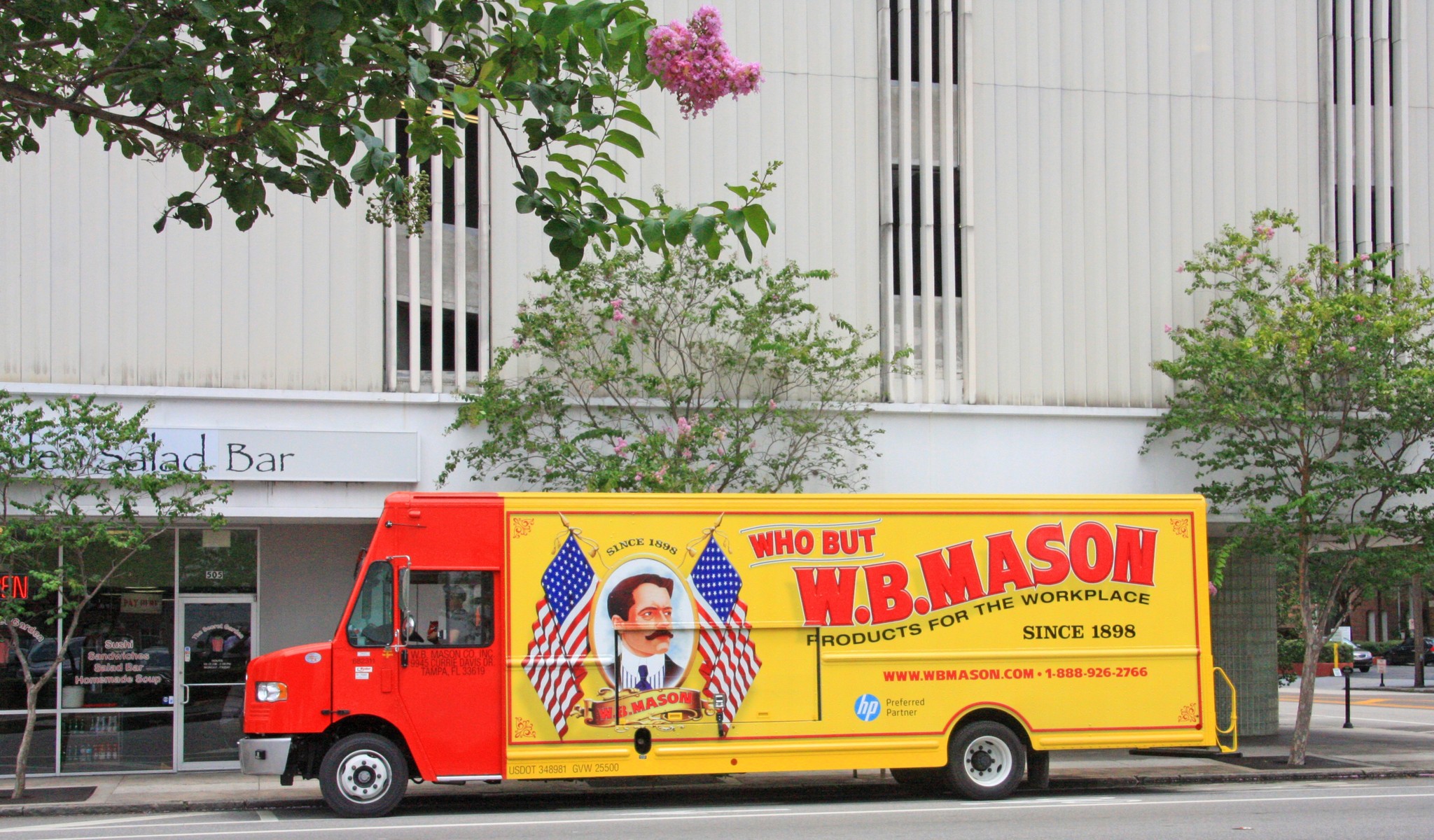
[{"xmin": 16, "ymin": 635, "xmax": 190, "ymax": 704}]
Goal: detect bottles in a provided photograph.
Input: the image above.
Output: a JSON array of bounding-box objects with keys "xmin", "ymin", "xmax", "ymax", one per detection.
[
  {"xmin": 107, "ymin": 716, "xmax": 118, "ymax": 733},
  {"xmin": 62, "ymin": 744, "xmax": 117, "ymax": 762},
  {"xmin": 74, "ymin": 719, "xmax": 78, "ymax": 735},
  {"xmin": 79, "ymin": 719, "xmax": 85, "ymax": 734},
  {"xmin": 90, "ymin": 716, "xmax": 105, "ymax": 734},
  {"xmin": 62, "ymin": 719, "xmax": 67, "ymax": 735},
  {"xmin": 67, "ymin": 719, "xmax": 73, "ymax": 735}
]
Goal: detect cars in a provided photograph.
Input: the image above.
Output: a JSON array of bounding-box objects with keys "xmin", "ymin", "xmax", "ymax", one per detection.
[
  {"xmin": 1381, "ymin": 637, "xmax": 1434, "ymax": 665},
  {"xmin": 1328, "ymin": 638, "xmax": 1373, "ymax": 672}
]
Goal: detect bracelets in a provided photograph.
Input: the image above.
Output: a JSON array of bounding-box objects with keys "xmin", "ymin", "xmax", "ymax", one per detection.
[{"xmin": 433, "ymin": 637, "xmax": 438, "ymax": 644}]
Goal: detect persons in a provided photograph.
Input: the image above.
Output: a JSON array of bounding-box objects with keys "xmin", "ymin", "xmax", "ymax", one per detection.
[
  {"xmin": 223, "ymin": 631, "xmax": 251, "ymax": 657},
  {"xmin": 426, "ymin": 585, "xmax": 475, "ymax": 645}
]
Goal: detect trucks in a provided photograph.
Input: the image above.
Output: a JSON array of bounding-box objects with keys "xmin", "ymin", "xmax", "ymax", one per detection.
[{"xmin": 236, "ymin": 489, "xmax": 1238, "ymax": 817}]
